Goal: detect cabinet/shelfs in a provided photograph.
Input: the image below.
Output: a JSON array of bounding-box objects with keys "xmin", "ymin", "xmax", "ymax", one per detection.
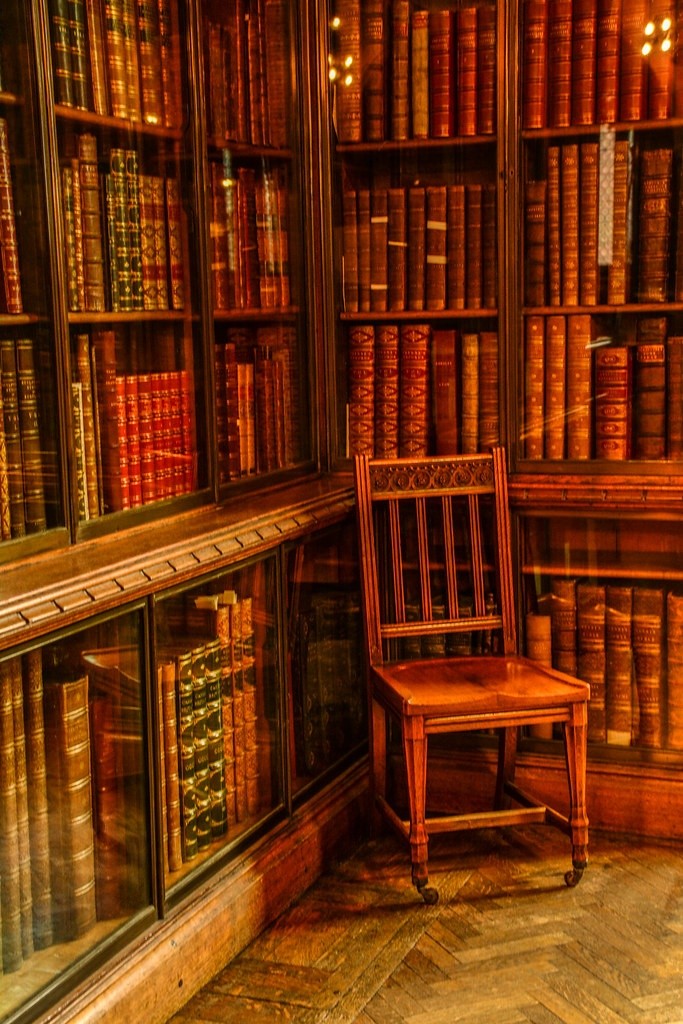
[{"xmin": 0, "ymin": 2, "xmax": 683, "ymax": 1023}]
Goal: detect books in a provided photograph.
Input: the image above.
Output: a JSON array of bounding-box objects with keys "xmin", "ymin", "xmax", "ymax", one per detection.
[{"xmin": 1, "ymin": 0, "xmax": 683, "ymax": 979}]
